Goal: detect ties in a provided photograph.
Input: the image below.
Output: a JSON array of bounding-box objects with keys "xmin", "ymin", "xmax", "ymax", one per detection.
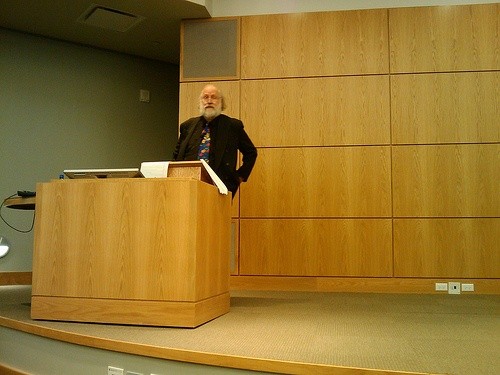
[{"xmin": 197, "ymin": 123, "xmax": 210, "ymax": 165}]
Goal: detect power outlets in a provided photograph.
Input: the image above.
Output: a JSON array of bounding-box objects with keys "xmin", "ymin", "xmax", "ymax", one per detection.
[
  {"xmin": 462, "ymin": 284, "xmax": 474, "ymax": 291},
  {"xmin": 436, "ymin": 283, "xmax": 447, "ymax": 291},
  {"xmin": 448, "ymin": 282, "xmax": 460, "ymax": 294}
]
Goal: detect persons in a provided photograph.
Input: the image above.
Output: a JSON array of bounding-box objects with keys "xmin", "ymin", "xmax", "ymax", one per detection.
[{"xmin": 173, "ymin": 84, "xmax": 258, "ymax": 202}]
